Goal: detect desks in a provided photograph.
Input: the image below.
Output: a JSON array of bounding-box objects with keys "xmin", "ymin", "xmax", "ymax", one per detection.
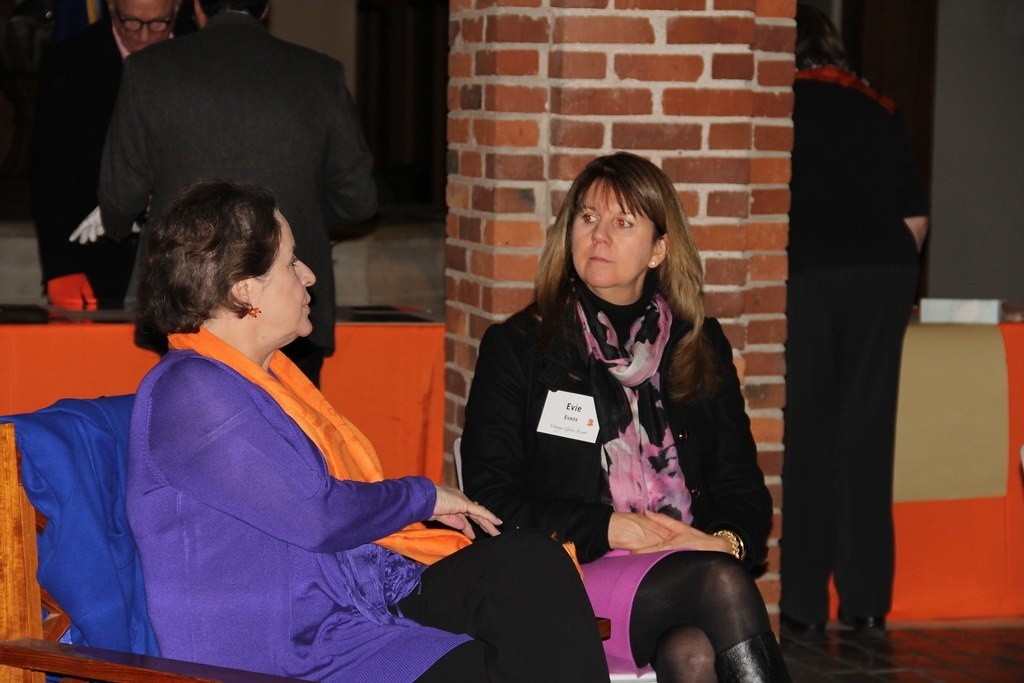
[{"xmin": 0, "ymin": 309, "xmax": 1024, "ymax": 629}]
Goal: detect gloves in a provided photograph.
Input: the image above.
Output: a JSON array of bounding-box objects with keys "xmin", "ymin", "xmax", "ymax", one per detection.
[
  {"xmin": 69, "ymin": 205, "xmax": 141, "ymax": 244},
  {"xmin": 47, "ymin": 273, "xmax": 97, "ymax": 324}
]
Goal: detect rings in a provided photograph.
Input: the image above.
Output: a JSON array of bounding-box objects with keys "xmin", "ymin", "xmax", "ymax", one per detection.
[{"xmin": 473, "ymin": 501, "xmax": 478, "ymax": 504}]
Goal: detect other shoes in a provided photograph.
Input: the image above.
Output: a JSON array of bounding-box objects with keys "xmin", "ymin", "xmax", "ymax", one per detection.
[
  {"xmin": 780, "ymin": 615, "xmax": 825, "ymax": 637},
  {"xmin": 839, "ymin": 616, "xmax": 886, "ymax": 632}
]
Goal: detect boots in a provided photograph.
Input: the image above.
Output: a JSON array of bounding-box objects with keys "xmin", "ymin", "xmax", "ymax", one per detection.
[{"xmin": 715, "ymin": 631, "xmax": 791, "ymax": 683}]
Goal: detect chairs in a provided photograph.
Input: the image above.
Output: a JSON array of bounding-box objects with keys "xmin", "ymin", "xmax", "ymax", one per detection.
[{"xmin": 0, "ymin": 394, "xmax": 316, "ymax": 683}]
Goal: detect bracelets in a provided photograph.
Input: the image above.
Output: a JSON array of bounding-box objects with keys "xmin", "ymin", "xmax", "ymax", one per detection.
[{"xmin": 713, "ymin": 530, "xmax": 744, "ymax": 559}]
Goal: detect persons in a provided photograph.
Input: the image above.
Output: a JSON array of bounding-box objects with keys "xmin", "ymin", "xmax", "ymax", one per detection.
[
  {"xmin": 459, "ymin": 152, "xmax": 793, "ymax": 683},
  {"xmin": 779, "ymin": 3, "xmax": 930, "ymax": 634},
  {"xmin": 122, "ymin": 178, "xmax": 611, "ymax": 683},
  {"xmin": 68, "ymin": 0, "xmax": 381, "ymax": 390},
  {"xmin": 28, "ymin": 0, "xmax": 183, "ymax": 311}
]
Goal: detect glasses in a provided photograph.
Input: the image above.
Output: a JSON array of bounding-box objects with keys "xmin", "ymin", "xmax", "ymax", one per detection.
[{"xmin": 113, "ymin": 3, "xmax": 176, "ymax": 32}]
